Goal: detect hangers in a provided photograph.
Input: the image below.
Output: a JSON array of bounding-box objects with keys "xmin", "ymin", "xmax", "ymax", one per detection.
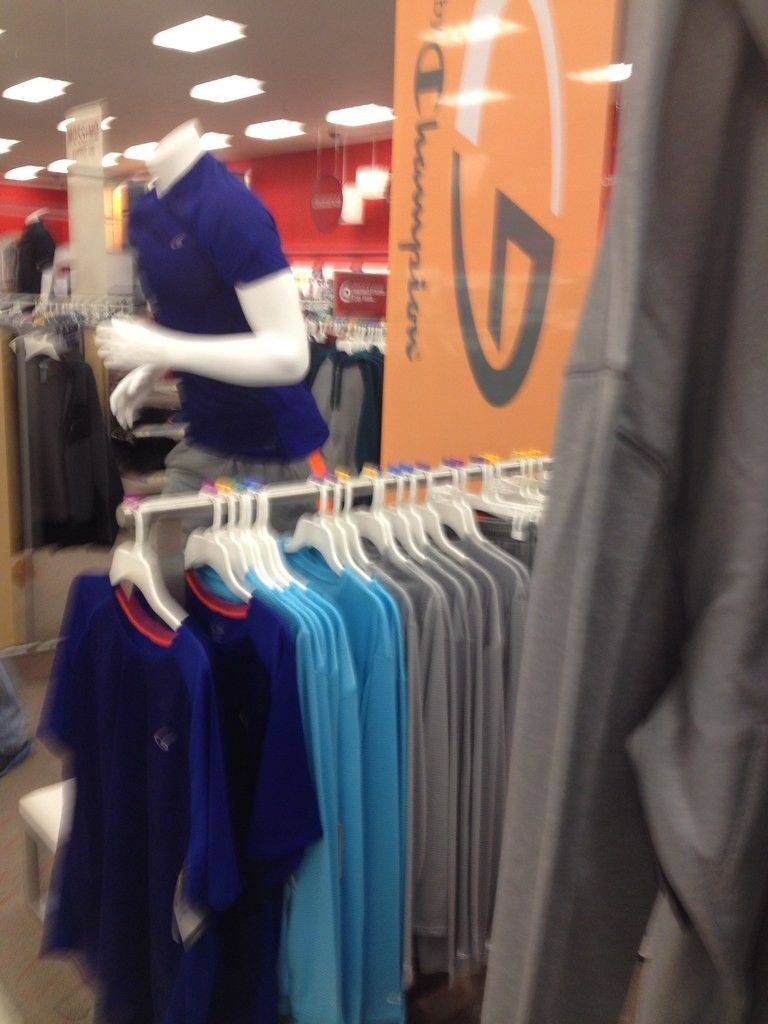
[
  {"xmin": 109, "ymin": 453, "xmax": 556, "ymax": 633},
  {"xmin": 301, "ymin": 319, "xmax": 386, "ymax": 355},
  {"xmin": 0, "ymin": 293, "xmax": 135, "ymax": 328}
]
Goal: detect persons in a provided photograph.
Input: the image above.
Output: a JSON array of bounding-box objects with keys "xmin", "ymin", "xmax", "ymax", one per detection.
[
  {"xmin": 93, "ymin": 120, "xmax": 344, "ymax": 607},
  {"xmin": 8, "ymin": 213, "xmax": 56, "ymax": 318}
]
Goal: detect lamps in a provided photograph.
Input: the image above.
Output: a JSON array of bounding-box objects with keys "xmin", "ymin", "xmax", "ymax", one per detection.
[{"xmin": 342, "ymin": 133, "xmax": 389, "ymax": 230}]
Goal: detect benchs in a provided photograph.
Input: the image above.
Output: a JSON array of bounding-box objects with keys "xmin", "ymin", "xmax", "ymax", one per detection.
[{"xmin": 19, "ymin": 776, "xmax": 71, "ymax": 925}]
